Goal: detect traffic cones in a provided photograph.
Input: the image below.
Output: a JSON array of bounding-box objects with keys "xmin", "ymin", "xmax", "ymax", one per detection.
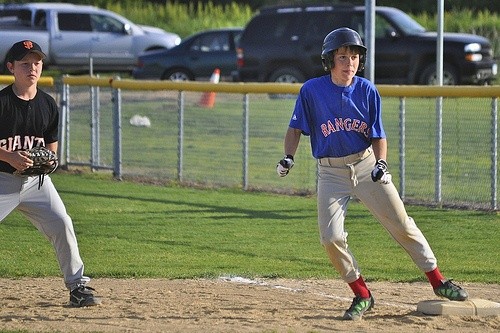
[{"xmin": 199, "ymin": 68, "xmax": 222, "ymax": 109}]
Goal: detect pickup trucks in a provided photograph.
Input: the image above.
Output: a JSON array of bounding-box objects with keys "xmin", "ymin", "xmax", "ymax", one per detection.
[{"xmin": 1, "ymin": 4, "xmax": 183, "ymax": 73}]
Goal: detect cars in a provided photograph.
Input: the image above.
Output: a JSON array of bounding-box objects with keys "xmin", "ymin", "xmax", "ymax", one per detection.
[{"xmin": 133, "ymin": 29, "xmax": 243, "ymax": 81}]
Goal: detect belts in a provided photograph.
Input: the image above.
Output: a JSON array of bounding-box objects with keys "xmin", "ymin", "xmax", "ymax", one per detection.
[{"xmin": 318, "ymin": 143, "xmax": 372, "ymax": 168}]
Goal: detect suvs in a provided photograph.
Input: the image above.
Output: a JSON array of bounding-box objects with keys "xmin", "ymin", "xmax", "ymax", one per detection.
[{"xmin": 231, "ymin": 5, "xmax": 497, "ymax": 99}]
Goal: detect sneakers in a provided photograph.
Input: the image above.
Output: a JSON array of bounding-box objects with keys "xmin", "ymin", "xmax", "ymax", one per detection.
[
  {"xmin": 343, "ymin": 290, "xmax": 375, "ymax": 320},
  {"xmin": 70, "ymin": 285, "xmax": 101, "ymax": 307},
  {"xmin": 434, "ymin": 278, "xmax": 468, "ymax": 301}
]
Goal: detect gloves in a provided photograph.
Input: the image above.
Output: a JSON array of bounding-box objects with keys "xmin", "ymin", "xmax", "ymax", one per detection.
[
  {"xmin": 276, "ymin": 155, "xmax": 295, "ymax": 177},
  {"xmin": 371, "ymin": 159, "xmax": 392, "ymax": 184}
]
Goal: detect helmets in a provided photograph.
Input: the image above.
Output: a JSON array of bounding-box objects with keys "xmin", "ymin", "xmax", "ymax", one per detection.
[{"xmin": 321, "ymin": 27, "xmax": 367, "ymax": 73}]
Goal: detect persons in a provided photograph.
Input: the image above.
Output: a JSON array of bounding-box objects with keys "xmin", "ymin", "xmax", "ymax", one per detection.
[
  {"xmin": 0, "ymin": 40, "xmax": 100, "ymax": 307},
  {"xmin": 276, "ymin": 27, "xmax": 468, "ymax": 321}
]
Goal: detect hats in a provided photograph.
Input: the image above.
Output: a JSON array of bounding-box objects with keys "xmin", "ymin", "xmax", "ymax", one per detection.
[{"xmin": 9, "ymin": 40, "xmax": 48, "ymax": 61}]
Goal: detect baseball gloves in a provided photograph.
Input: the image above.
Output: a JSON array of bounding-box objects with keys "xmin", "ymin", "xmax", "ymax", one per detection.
[{"xmin": 20, "ymin": 150, "xmax": 59, "ymax": 177}]
